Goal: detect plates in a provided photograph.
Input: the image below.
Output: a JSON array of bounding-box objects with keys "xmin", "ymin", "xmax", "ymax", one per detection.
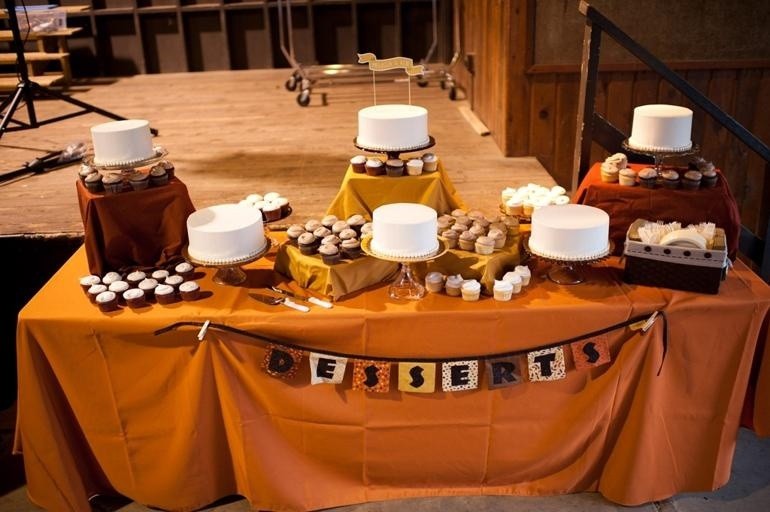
[
  {"xmin": 621, "ymin": 137, "xmax": 702, "ymax": 158},
  {"xmin": 353, "ymin": 134, "xmax": 436, "ymax": 152},
  {"xmin": 83, "ymin": 148, "xmax": 169, "ymax": 168},
  {"xmin": 181, "ymin": 237, "xmax": 271, "ymax": 268},
  {"xmin": 522, "ymin": 237, "xmax": 617, "ymax": 263},
  {"xmin": 499, "ymin": 202, "xmax": 533, "ymax": 222},
  {"xmin": 263, "ymin": 206, "xmax": 292, "ymax": 223},
  {"xmin": 360, "ymin": 233, "xmax": 448, "ymax": 262}
]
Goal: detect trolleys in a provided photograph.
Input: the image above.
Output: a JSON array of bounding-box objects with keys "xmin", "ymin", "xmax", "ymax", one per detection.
[{"xmin": 276, "ymin": 0, "xmax": 464, "ymax": 107}]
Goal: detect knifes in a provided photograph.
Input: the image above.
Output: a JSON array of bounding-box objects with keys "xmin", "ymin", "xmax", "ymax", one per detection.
[
  {"xmin": 247, "ymin": 292, "xmax": 309, "ymax": 312},
  {"xmin": 264, "ymin": 282, "xmax": 335, "ymax": 309}
]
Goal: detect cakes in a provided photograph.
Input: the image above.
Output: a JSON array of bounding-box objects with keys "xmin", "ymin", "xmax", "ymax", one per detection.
[
  {"xmin": 385, "ymin": 158, "xmax": 404, "ymax": 177},
  {"xmin": 126, "ymin": 269, "xmax": 147, "ymax": 288},
  {"xmin": 425, "ymin": 271, "xmax": 443, "ymax": 293},
  {"xmin": 406, "ymin": 159, "xmax": 424, "ymax": 177},
  {"xmin": 317, "ymin": 242, "xmax": 340, "ymax": 265},
  {"xmin": 347, "ymin": 214, "xmax": 365, "ymax": 235},
  {"xmin": 515, "ymin": 265, "xmax": 532, "ymax": 288},
  {"xmin": 79, "ymin": 274, "xmax": 101, "ymax": 295},
  {"xmin": 699, "ymin": 161, "xmax": 715, "ymax": 172},
  {"xmin": 101, "ymin": 173, "xmax": 124, "ymax": 195},
  {"xmin": 95, "ymin": 290, "xmax": 118, "ymax": 312},
  {"xmin": 364, "ymin": 158, "xmax": 384, "ymax": 176},
  {"xmin": 361, "ymin": 222, "xmax": 372, "ymax": 239},
  {"xmin": 262, "ymin": 203, "xmax": 281, "ymax": 221},
  {"xmin": 702, "ymin": 170, "xmax": 718, "ymax": 188},
  {"xmin": 151, "ymin": 269, "xmax": 169, "ymax": 284},
  {"xmin": 450, "ymin": 223, "xmax": 468, "ymax": 235},
  {"xmin": 122, "ymin": 288, "xmax": 146, "ymax": 308},
  {"xmin": 600, "ymin": 161, "xmax": 619, "ymax": 183},
  {"xmin": 271, "ymin": 197, "xmax": 289, "ymax": 218},
  {"xmin": 305, "ymin": 219, "xmax": 322, "ymax": 234},
  {"xmin": 688, "ymin": 157, "xmax": 704, "ymax": 171},
  {"xmin": 618, "ymin": 168, "xmax": 637, "ymax": 186},
  {"xmin": 157, "ymin": 160, "xmax": 174, "ymax": 179},
  {"xmin": 451, "ymin": 209, "xmax": 466, "ymax": 218},
  {"xmin": 370, "ymin": 202, "xmax": 439, "ymax": 259},
  {"xmin": 500, "ymin": 182, "xmax": 571, "ymax": 218},
  {"xmin": 488, "ymin": 229, "xmax": 505, "ymax": 247},
  {"xmin": 455, "ymin": 215, "xmax": 471, "ymax": 227},
  {"xmin": 356, "ymin": 103, "xmax": 430, "ymax": 151},
  {"xmin": 321, "ymin": 234, "xmax": 340, "ymax": 249},
  {"xmin": 484, "ymin": 213, "xmax": 500, "ymax": 225},
  {"xmin": 493, "ymin": 279, "xmax": 514, "ymax": 302},
  {"xmin": 245, "ymin": 193, "xmax": 263, "ymax": 202},
  {"xmin": 683, "ymin": 170, "xmax": 702, "ymax": 191},
  {"xmin": 102, "ymin": 272, "xmax": 123, "ymax": 287},
  {"xmin": 442, "ymin": 229, "xmax": 458, "ymax": 249},
  {"xmin": 467, "ymin": 210, "xmax": 484, "ymax": 222},
  {"xmin": 239, "ymin": 199, "xmax": 254, "ymax": 206},
  {"xmin": 313, "ymin": 226, "xmax": 331, "ymax": 246},
  {"xmin": 605, "ymin": 156, "xmax": 623, "ymax": 179},
  {"xmin": 503, "ymin": 215, "xmax": 521, "ymax": 236},
  {"xmin": 502, "ymin": 271, "xmax": 522, "ymax": 294},
  {"xmin": 469, "ymin": 222, "xmax": 484, "ymax": 237},
  {"xmin": 78, "ymin": 165, "xmax": 98, "ymax": 188},
  {"xmin": 612, "ymin": 152, "xmax": 627, "ymax": 169},
  {"xmin": 474, "ymin": 235, "xmax": 495, "ymax": 255},
  {"xmin": 350, "ymin": 154, "xmax": 368, "ymax": 173},
  {"xmin": 150, "ymin": 165, "xmax": 169, "ymax": 187},
  {"xmin": 178, "ymin": 281, "xmax": 200, "ymax": 301},
  {"xmin": 339, "ymin": 228, "xmax": 359, "ymax": 241},
  {"xmin": 458, "ymin": 230, "xmax": 476, "ymax": 251},
  {"xmin": 87, "ymin": 283, "xmax": 108, "ymax": 304},
  {"xmin": 628, "ymin": 104, "xmax": 693, "ymax": 153},
  {"xmin": 321, "ymin": 214, "xmax": 338, "ymax": 230},
  {"xmin": 84, "ymin": 172, "xmax": 103, "ymax": 193},
  {"xmin": 186, "ymin": 204, "xmax": 268, "ymax": 265},
  {"xmin": 164, "ymin": 274, "xmax": 184, "ymax": 295},
  {"xmin": 661, "ymin": 169, "xmax": 680, "ymax": 190},
  {"xmin": 528, "ymin": 203, "xmax": 610, "ymax": 261},
  {"xmin": 420, "ymin": 153, "xmax": 438, "ymax": 172},
  {"xmin": 254, "ymin": 201, "xmax": 269, "ymax": 220},
  {"xmin": 174, "ymin": 262, "xmax": 194, "ymax": 282},
  {"xmin": 153, "ymin": 284, "xmax": 176, "ymax": 305},
  {"xmin": 108, "ymin": 280, "xmax": 129, "ymax": 304},
  {"xmin": 89, "ymin": 118, "xmax": 155, "ymax": 167},
  {"xmin": 488, "ymin": 222, "xmax": 507, "ymax": 241},
  {"xmin": 286, "ymin": 226, "xmax": 305, "ymax": 247},
  {"xmin": 264, "ymin": 191, "xmax": 280, "ymax": 203},
  {"xmin": 638, "ymin": 167, "xmax": 658, "ymax": 189},
  {"xmin": 339, "ymin": 238, "xmax": 360, "ymax": 259},
  {"xmin": 436, "ymin": 216, "xmax": 449, "ymax": 235},
  {"xmin": 460, "ymin": 278, "xmax": 481, "ymax": 302},
  {"xmin": 297, "ymin": 232, "xmax": 318, "ymax": 255},
  {"xmin": 127, "ymin": 169, "xmax": 150, "ymax": 191},
  {"xmin": 445, "ymin": 273, "xmax": 463, "ymax": 296},
  {"xmin": 138, "ymin": 277, "xmax": 158, "ymax": 302},
  {"xmin": 332, "ymin": 220, "xmax": 350, "ymax": 237}
]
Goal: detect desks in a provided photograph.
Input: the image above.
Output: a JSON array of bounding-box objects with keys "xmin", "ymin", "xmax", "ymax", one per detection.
[{"xmin": 13, "ymin": 240, "xmax": 770, "ymax": 512}]
